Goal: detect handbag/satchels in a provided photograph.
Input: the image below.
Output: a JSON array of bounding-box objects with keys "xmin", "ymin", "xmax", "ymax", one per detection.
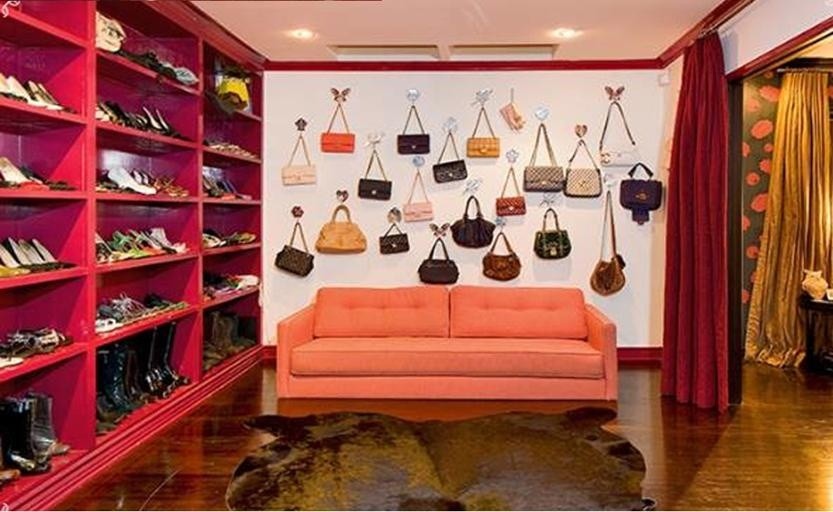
[
  {"xmin": 321, "ymin": 133, "xmax": 354, "ymax": 153},
  {"xmin": 276, "ymin": 245, "xmax": 313, "ymax": 276},
  {"xmin": 219, "ymin": 77, "xmax": 250, "ymax": 110},
  {"xmin": 175, "ymin": 65, "xmax": 197, "ymax": 87},
  {"xmin": 484, "ymin": 252, "xmax": 521, "ymax": 281},
  {"xmin": 404, "ymin": 203, "xmax": 434, "ymax": 222},
  {"xmin": 398, "ymin": 134, "xmax": 430, "ymax": 154},
  {"xmin": 621, "ymin": 179, "xmax": 662, "ymax": 225},
  {"xmin": 501, "ymin": 103, "xmax": 526, "ymax": 130},
  {"xmin": 359, "ymin": 179, "xmax": 391, "ymax": 199},
  {"xmin": 524, "ymin": 166, "xmax": 564, "ymax": 192},
  {"xmin": 316, "ymin": 206, "xmax": 366, "ymax": 253},
  {"xmin": 418, "ymin": 259, "xmax": 458, "ymax": 283},
  {"xmin": 565, "ymin": 167, "xmax": 602, "ymax": 198},
  {"xmin": 283, "ymin": 165, "xmax": 315, "ymax": 185},
  {"xmin": 591, "ymin": 256, "xmax": 626, "ymax": 295},
  {"xmin": 496, "ymin": 196, "xmax": 525, "ymax": 215},
  {"xmin": 451, "ymin": 219, "xmax": 495, "ymax": 248},
  {"xmin": 600, "ymin": 148, "xmax": 638, "ymax": 166},
  {"xmin": 96, "ymin": 9, "xmax": 126, "ymax": 53},
  {"xmin": 467, "ymin": 137, "xmax": 500, "ymax": 158},
  {"xmin": 379, "ymin": 223, "xmax": 409, "ymax": 254},
  {"xmin": 534, "ymin": 230, "xmax": 571, "ymax": 258},
  {"xmin": 433, "ymin": 160, "xmax": 467, "ymax": 182}
]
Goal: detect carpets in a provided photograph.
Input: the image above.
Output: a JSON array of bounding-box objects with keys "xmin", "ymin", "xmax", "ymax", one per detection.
[{"xmin": 224, "ymin": 406, "xmax": 656, "ymax": 511}]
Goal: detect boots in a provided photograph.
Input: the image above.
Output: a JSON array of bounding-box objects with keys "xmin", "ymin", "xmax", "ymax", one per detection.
[
  {"xmin": 0, "ymin": 390, "xmax": 70, "ymax": 484},
  {"xmin": 96, "ymin": 322, "xmax": 191, "ymax": 434},
  {"xmin": 203, "ymin": 310, "xmax": 256, "ymax": 372}
]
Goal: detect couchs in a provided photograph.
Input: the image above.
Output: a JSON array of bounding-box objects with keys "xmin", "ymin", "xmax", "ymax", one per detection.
[{"xmin": 276, "ymin": 283, "xmax": 618, "ymax": 402}]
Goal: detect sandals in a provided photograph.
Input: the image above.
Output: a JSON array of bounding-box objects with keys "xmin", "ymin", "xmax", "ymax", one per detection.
[
  {"xmin": 95, "ymin": 291, "xmax": 189, "ymax": 334},
  {"xmin": 0, "ymin": 326, "xmax": 73, "ymax": 368},
  {"xmin": 202, "ymin": 227, "xmax": 256, "ymax": 248},
  {"xmin": 204, "ymin": 270, "xmax": 259, "ymax": 300},
  {"xmin": 204, "ymin": 138, "xmax": 257, "ymax": 158}
]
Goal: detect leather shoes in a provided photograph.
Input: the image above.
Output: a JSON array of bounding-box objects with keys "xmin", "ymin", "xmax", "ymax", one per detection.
[
  {"xmin": 0, "ymin": 73, "xmax": 71, "ymax": 112},
  {"xmin": 203, "ymin": 175, "xmax": 253, "ymax": 200},
  {"xmin": 95, "ymin": 228, "xmax": 185, "ymax": 263},
  {"xmin": 96, "ymin": 99, "xmax": 186, "ymax": 137},
  {"xmin": 96, "ymin": 168, "xmax": 189, "ymax": 197},
  {"xmin": 122, "ymin": 46, "xmax": 177, "ymax": 80},
  {"xmin": 0, "ymin": 157, "xmax": 77, "ymax": 190},
  {"xmin": 1, "ymin": 236, "xmax": 76, "ymax": 276}
]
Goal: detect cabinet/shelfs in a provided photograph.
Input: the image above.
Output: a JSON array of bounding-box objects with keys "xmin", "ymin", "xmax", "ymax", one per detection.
[
  {"xmin": 799, "ymin": 290, "xmax": 833, "ymax": 376},
  {"xmin": 0, "ymin": 0, "xmax": 262, "ymax": 511}
]
